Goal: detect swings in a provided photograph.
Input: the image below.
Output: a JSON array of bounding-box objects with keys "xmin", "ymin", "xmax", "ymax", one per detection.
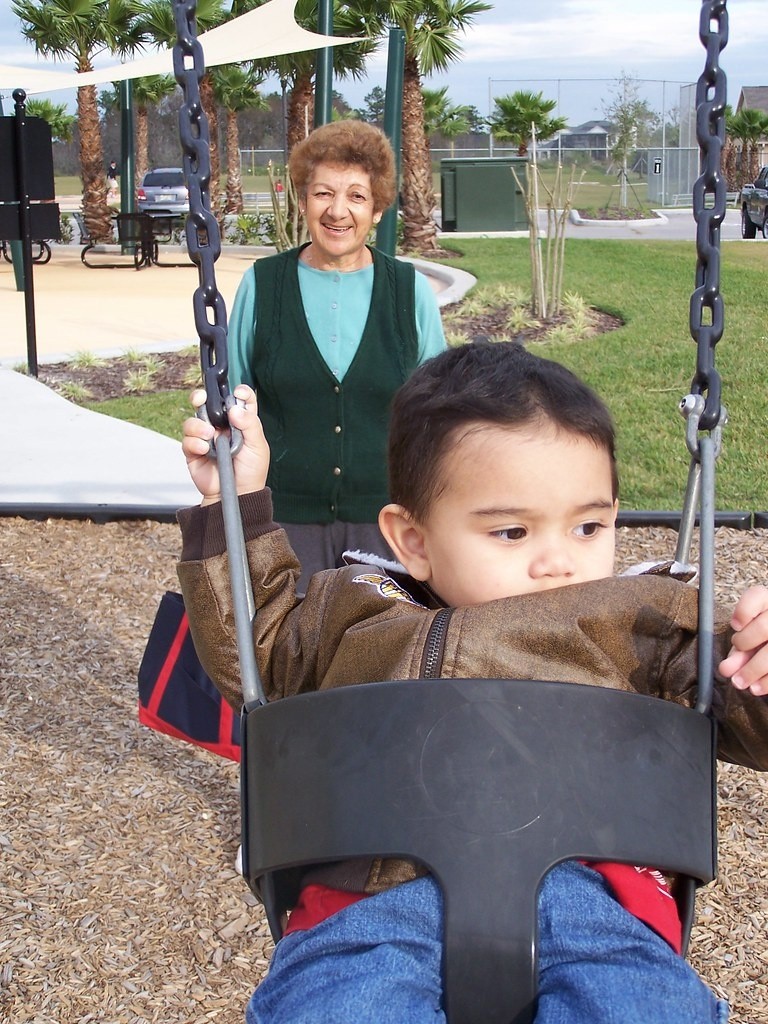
[{"xmin": 167, "ymin": 0, "xmax": 729, "ymax": 1024}]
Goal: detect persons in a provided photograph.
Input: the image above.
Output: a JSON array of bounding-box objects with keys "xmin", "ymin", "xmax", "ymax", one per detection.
[
  {"xmin": 106, "ymin": 160, "xmax": 117, "ymax": 198},
  {"xmin": 218, "ymin": 122, "xmax": 448, "ymax": 593},
  {"xmin": 173, "ymin": 341, "xmax": 768, "ymax": 1024}
]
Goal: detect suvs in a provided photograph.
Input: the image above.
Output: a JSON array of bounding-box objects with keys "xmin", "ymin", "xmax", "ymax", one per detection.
[{"xmin": 137, "ymin": 167, "xmax": 190, "ymax": 217}]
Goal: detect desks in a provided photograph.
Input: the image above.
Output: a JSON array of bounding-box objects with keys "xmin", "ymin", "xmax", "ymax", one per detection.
[{"xmin": 110, "ymin": 213, "xmax": 180, "ymax": 266}]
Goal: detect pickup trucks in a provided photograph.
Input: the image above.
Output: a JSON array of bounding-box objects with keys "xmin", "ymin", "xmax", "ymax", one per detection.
[{"xmin": 740, "ymin": 165, "xmax": 768, "ymax": 238}]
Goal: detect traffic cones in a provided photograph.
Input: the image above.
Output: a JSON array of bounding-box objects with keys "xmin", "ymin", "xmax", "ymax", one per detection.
[{"xmin": 276, "ymin": 179, "xmax": 282, "ymax": 192}]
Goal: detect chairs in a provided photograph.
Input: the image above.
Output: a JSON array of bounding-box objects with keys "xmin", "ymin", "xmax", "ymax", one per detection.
[{"xmin": 73, "ymin": 208, "xmax": 173, "ymax": 271}]
